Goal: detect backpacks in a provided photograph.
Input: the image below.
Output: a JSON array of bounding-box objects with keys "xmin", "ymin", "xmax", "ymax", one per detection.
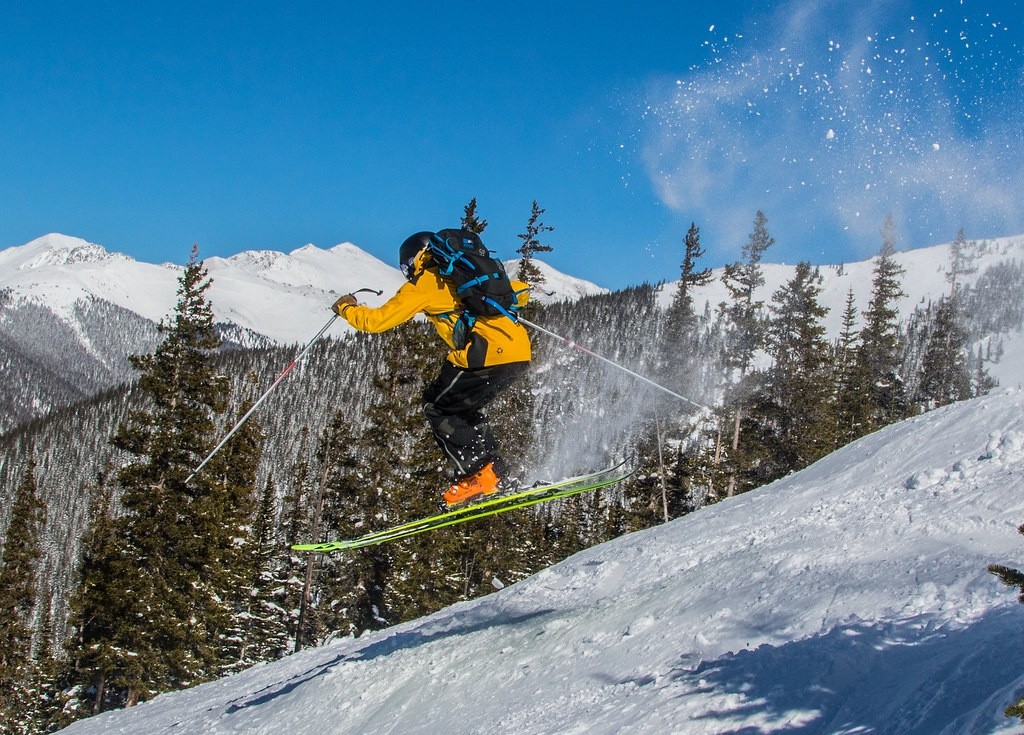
[{"xmin": 423, "ymin": 228, "xmax": 518, "ymax": 316}]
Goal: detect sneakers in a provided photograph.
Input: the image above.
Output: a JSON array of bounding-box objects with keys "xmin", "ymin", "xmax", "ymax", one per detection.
[{"xmin": 443, "ymin": 462, "xmax": 500, "ymax": 507}]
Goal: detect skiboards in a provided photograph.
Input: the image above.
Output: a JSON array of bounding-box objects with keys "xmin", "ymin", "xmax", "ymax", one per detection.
[{"xmin": 289, "ymin": 448, "xmax": 643, "ymax": 555}]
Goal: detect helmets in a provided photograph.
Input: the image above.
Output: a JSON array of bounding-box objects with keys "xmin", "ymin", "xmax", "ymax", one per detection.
[{"xmin": 399, "ymin": 231, "xmax": 434, "ymax": 280}]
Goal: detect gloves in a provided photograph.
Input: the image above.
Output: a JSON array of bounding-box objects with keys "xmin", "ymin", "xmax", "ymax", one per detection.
[{"xmin": 331, "ymin": 294, "xmax": 357, "ymax": 316}]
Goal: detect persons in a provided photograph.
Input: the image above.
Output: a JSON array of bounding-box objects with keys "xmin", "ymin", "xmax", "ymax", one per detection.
[{"xmin": 331, "ymin": 231, "xmax": 532, "ymax": 510}]
